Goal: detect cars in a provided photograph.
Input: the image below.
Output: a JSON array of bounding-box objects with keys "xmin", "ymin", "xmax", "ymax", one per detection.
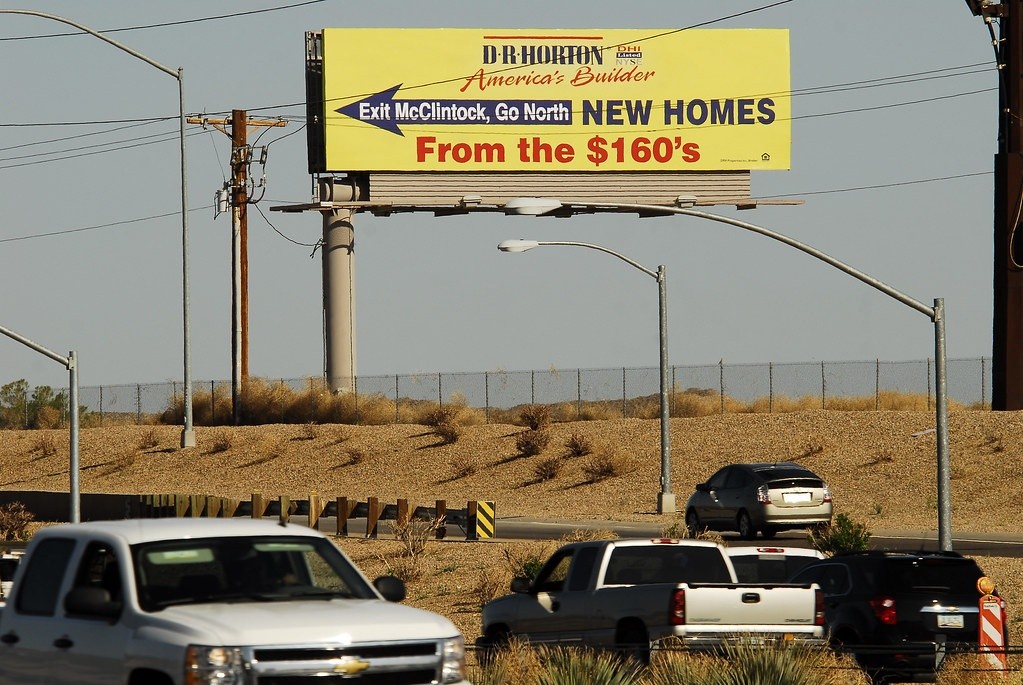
[
  {"xmin": 0, "ymin": 551, "xmax": 30, "ymax": 602},
  {"xmin": 685, "ymin": 462, "xmax": 835, "ymax": 540}
]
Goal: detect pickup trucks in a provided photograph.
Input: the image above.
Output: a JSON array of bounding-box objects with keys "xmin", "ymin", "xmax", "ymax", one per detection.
[
  {"xmin": 475, "ymin": 536, "xmax": 823, "ymax": 667},
  {"xmin": 727, "ymin": 548, "xmax": 824, "ymax": 593},
  {"xmin": 0, "ymin": 516, "xmax": 473, "ymax": 685}
]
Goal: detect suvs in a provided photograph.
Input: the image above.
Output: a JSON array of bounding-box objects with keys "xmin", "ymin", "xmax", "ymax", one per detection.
[{"xmin": 787, "ymin": 550, "xmax": 1008, "ymax": 685}]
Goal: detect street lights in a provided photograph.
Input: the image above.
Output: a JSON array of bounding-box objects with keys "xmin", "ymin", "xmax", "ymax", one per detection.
[
  {"xmin": 499, "ymin": 238, "xmax": 671, "ymax": 493},
  {"xmin": 503, "ymin": 194, "xmax": 952, "ymax": 553}
]
